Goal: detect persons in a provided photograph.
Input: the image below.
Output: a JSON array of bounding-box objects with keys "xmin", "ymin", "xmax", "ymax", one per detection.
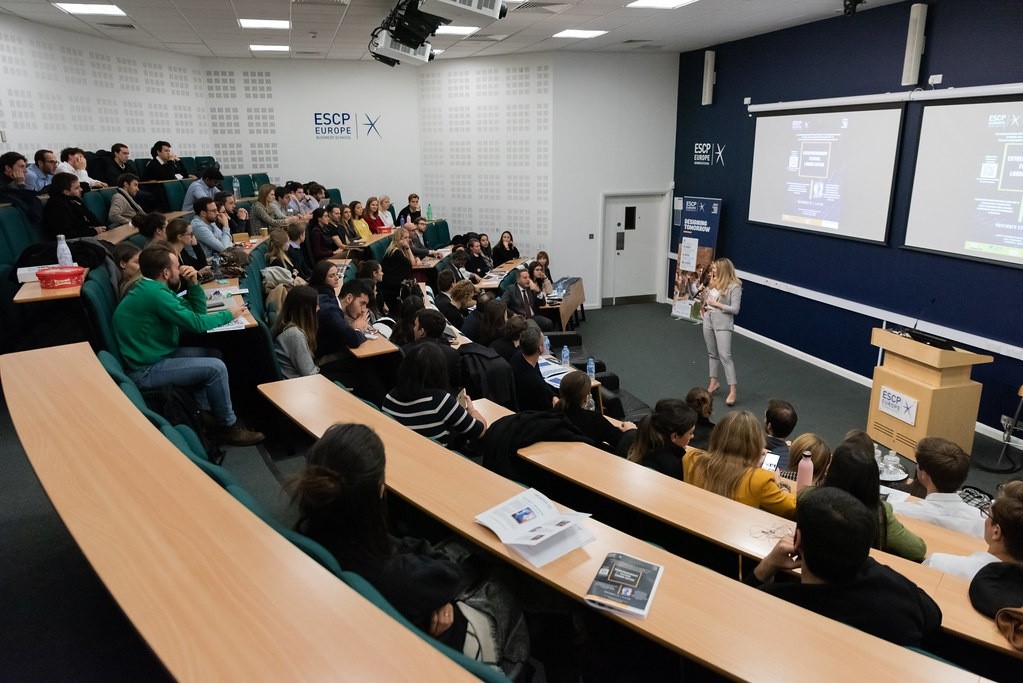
[
  {"xmin": 114, "ymin": 245, "xmax": 266, "ymax": 446},
  {"xmin": 683, "ymin": 410, "xmax": 797, "ymax": 517},
  {"xmin": 673, "ymin": 264, "xmax": 712, "ymax": 302},
  {"xmin": 118, "ymin": 193, "xmax": 251, "ymax": 304},
  {"xmin": 780, "ymin": 429, "xmax": 926, "ymax": 563},
  {"xmin": 698, "ymin": 259, "xmax": 741, "ymax": 405},
  {"xmin": 745, "ymin": 485, "xmax": 942, "ymax": 646},
  {"xmin": 765, "ymin": 399, "xmax": 798, "ymax": 469},
  {"xmin": 628, "ymin": 399, "xmax": 696, "ymax": 481},
  {"xmin": 291, "ymin": 424, "xmax": 530, "ymax": 679},
  {"xmin": 182, "ymin": 168, "xmax": 224, "ymax": 221},
  {"xmin": 921, "ymin": 481, "xmax": 1023, "ymax": 576},
  {"xmin": 251, "ymin": 180, "xmax": 637, "ymax": 458},
  {"xmin": 686, "ymin": 387, "xmax": 716, "ymax": 450},
  {"xmin": 893, "ymin": 437, "xmax": 988, "ymax": 538},
  {"xmin": 0, "ymin": 141, "xmax": 197, "ymax": 238}
]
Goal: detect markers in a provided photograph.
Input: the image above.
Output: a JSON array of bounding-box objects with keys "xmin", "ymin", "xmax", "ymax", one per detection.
[{"xmin": 244, "ymin": 301, "xmax": 250, "ymax": 306}]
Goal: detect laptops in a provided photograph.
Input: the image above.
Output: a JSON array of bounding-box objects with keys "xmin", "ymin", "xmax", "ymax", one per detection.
[
  {"xmin": 907, "ymin": 329, "xmax": 956, "ymax": 352},
  {"xmin": 319, "ymin": 199, "xmax": 330, "ymax": 209}
]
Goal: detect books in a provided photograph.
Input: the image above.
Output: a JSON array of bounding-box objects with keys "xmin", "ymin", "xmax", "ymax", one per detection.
[
  {"xmin": 584, "ymin": 552, "xmax": 664, "ymax": 617},
  {"xmin": 475, "ymin": 488, "xmax": 594, "ymax": 568}
]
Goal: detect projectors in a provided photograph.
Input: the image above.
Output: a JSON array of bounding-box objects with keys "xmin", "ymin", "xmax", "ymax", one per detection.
[
  {"xmin": 418, "ymin": 0, "xmax": 508, "ymax": 29},
  {"xmin": 371, "ymin": 28, "xmax": 435, "ymax": 66}
]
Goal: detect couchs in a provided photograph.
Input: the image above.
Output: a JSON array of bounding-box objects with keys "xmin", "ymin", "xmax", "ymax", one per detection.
[
  {"xmin": 542, "ymin": 331, "xmax": 607, "ymax": 374},
  {"xmin": 590, "ymin": 371, "xmax": 654, "ymax": 423}
]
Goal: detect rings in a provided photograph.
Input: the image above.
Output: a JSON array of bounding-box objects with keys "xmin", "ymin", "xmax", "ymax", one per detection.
[{"xmin": 445, "ymin": 614, "xmax": 450, "ymax": 616}]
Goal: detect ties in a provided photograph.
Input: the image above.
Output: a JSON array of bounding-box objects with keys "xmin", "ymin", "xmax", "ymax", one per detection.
[{"xmin": 523, "ymin": 290, "xmax": 531, "ymax": 319}]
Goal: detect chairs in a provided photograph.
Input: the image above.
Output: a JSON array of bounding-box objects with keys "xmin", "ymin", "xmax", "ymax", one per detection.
[{"xmin": 1, "ymin": 156, "xmax": 1023, "ymax": 683}]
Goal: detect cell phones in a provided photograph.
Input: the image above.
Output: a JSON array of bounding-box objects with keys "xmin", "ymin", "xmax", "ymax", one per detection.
[{"xmin": 761, "ymin": 452, "xmax": 781, "ymax": 472}]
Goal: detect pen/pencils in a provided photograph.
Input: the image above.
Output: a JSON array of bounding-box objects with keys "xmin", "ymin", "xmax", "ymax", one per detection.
[{"xmin": 234, "ymin": 294, "xmax": 244, "ymax": 295}]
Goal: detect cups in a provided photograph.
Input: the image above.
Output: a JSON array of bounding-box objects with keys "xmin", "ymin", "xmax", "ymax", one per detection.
[{"xmin": 260, "ymin": 228, "xmax": 268, "ymax": 237}]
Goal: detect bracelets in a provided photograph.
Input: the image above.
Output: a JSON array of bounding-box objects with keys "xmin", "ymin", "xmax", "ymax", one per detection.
[{"xmin": 406, "ymin": 246, "xmax": 410, "ymax": 250}]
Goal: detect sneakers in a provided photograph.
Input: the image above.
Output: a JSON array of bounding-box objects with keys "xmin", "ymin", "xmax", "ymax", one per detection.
[{"xmin": 226, "ymin": 424, "xmax": 264, "ymax": 446}]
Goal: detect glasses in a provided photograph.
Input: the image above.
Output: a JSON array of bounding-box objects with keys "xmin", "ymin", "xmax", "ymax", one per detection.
[
  {"xmin": 183, "ymin": 232, "xmax": 193, "ymax": 237},
  {"xmin": 207, "ymin": 209, "xmax": 216, "ymax": 212},
  {"xmin": 534, "ymin": 269, "xmax": 543, "ymax": 271},
  {"xmin": 980, "ymin": 503, "xmax": 994, "ymax": 520},
  {"xmin": 418, "ymin": 222, "xmax": 427, "ymax": 225},
  {"xmin": 46, "ymin": 160, "xmax": 59, "ymax": 165}
]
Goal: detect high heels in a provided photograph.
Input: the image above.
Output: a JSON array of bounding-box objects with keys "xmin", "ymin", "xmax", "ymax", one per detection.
[
  {"xmin": 726, "ymin": 394, "xmax": 736, "ymax": 404},
  {"xmin": 708, "ymin": 383, "xmax": 720, "ymax": 393}
]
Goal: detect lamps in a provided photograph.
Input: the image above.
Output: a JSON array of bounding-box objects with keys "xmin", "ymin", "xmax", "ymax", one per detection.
[
  {"xmin": 902, "ymin": 4, "xmax": 927, "ymax": 84},
  {"xmin": 701, "ymin": 49, "xmax": 715, "ymax": 105}
]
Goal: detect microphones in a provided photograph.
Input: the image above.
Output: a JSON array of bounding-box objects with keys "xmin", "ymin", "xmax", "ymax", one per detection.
[
  {"xmin": 692, "ymin": 280, "xmax": 709, "ymax": 299},
  {"xmin": 913, "ymin": 297, "xmax": 936, "ymax": 330}
]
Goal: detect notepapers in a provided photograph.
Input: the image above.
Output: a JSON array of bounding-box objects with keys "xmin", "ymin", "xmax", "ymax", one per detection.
[{"xmin": 218, "ymin": 280, "xmax": 229, "ymax": 285}]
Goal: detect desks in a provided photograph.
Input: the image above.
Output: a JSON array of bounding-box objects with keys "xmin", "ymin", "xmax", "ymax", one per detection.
[
  {"xmin": 539, "ymin": 277, "xmax": 586, "ymax": 331},
  {"xmin": 344, "ymin": 219, "xmax": 434, "ymax": 259},
  {"xmin": 324, "ymin": 258, "xmax": 399, "ymax": 355},
  {"xmin": 250, "ymin": 374, "xmax": 996, "ymax": 683},
  {"xmin": 197, "ymin": 231, "xmax": 272, "ymax": 334},
  {"xmin": 476, "ymin": 258, "xmax": 530, "ymax": 289},
  {"xmin": 514, "ymin": 438, "xmax": 1021, "ymax": 660},
  {"xmin": 0, "ymin": 341, "xmax": 485, "ymax": 683},
  {"xmin": 0, "ymin": 178, "xmax": 119, "ymax": 208},
  {"xmin": 13, "ymin": 210, "xmax": 196, "ymax": 302},
  {"xmin": 235, "ymin": 196, "xmax": 259, "ymax": 202},
  {"xmin": 412, "ymin": 281, "xmax": 472, "ymax": 349},
  {"xmin": 552, "ymin": 396, "xmax": 990, "ymax": 558},
  {"xmin": 138, "ymin": 177, "xmax": 199, "ymax": 184},
  {"xmin": 412, "ymin": 245, "xmax": 457, "ymax": 283}
]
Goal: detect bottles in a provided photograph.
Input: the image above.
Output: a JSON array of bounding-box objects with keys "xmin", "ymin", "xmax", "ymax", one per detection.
[
  {"xmin": 587, "ymin": 358, "xmax": 595, "ymax": 382},
  {"xmin": 233, "ymin": 177, "xmax": 241, "ymax": 201},
  {"xmin": 557, "ymin": 283, "xmax": 563, "ymax": 298},
  {"xmin": 56, "ymin": 235, "xmax": 73, "ymax": 266},
  {"xmin": 561, "ymin": 345, "xmax": 569, "ymax": 369},
  {"xmin": 407, "ymin": 213, "xmax": 411, "ymax": 223},
  {"xmin": 871, "ymin": 443, "xmax": 900, "ymax": 477},
  {"xmin": 544, "ymin": 336, "xmax": 550, "ymax": 358},
  {"xmin": 400, "ymin": 215, "xmax": 405, "ymax": 228},
  {"xmin": 427, "ymin": 204, "xmax": 432, "ymax": 220},
  {"xmin": 796, "ymin": 451, "xmax": 814, "ymax": 493}
]
